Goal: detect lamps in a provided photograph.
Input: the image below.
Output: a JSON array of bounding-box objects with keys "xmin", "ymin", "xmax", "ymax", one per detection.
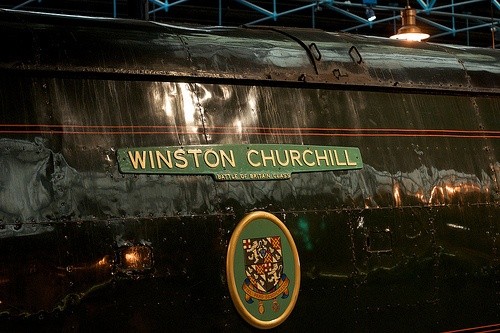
[{"xmin": 388, "ymin": 1, "xmax": 431, "ymax": 43}]
